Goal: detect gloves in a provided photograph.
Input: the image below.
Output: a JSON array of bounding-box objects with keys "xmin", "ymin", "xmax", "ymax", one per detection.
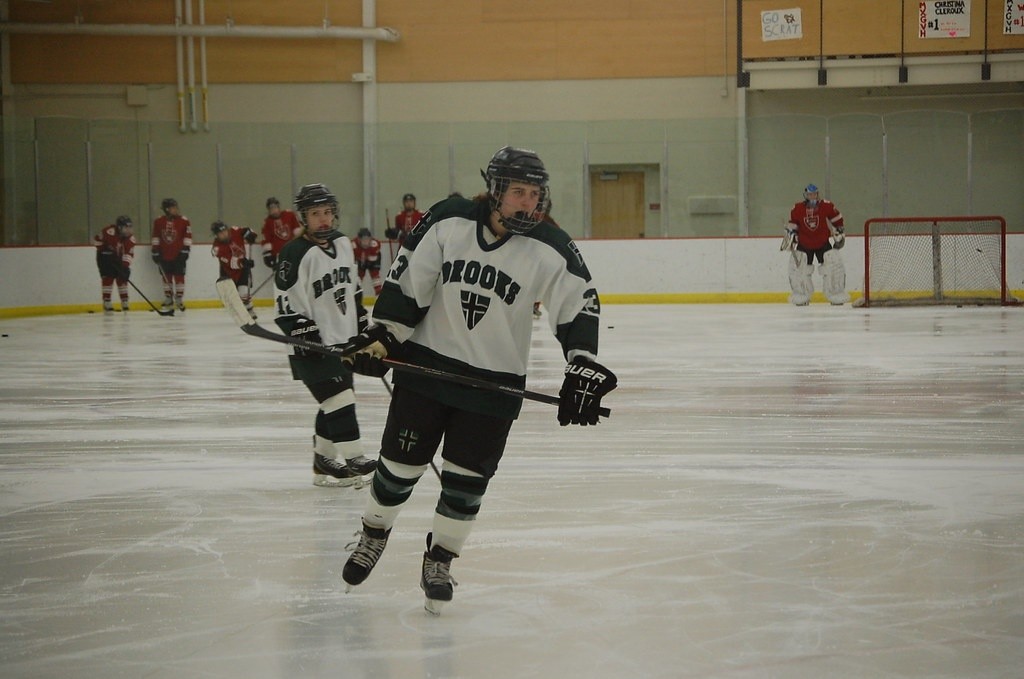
[
  {"xmin": 262, "ymin": 251, "xmax": 275, "ymax": 267},
  {"xmin": 242, "ymin": 229, "xmax": 258, "ymax": 247},
  {"xmin": 385, "ymin": 228, "xmax": 399, "ymax": 240},
  {"xmin": 153, "ymin": 246, "xmax": 162, "ymax": 264},
  {"xmin": 241, "ymin": 258, "xmax": 255, "ymax": 270},
  {"xmin": 558, "ymin": 356, "xmax": 619, "ymax": 427},
  {"xmin": 100, "ymin": 250, "xmax": 112, "ymax": 267},
  {"xmin": 121, "ymin": 268, "xmax": 130, "ymax": 282},
  {"xmin": 339, "ymin": 323, "xmax": 397, "ymax": 373},
  {"xmin": 178, "ymin": 248, "xmax": 190, "ymax": 263}
]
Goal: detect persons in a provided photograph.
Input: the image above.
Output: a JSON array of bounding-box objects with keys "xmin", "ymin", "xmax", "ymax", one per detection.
[
  {"xmin": 351, "ymin": 228, "xmax": 381, "ymax": 297},
  {"xmin": 385, "ymin": 193, "xmax": 426, "ymax": 246},
  {"xmin": 780, "ymin": 184, "xmax": 850, "ymax": 305},
  {"xmin": 211, "ymin": 222, "xmax": 258, "ymax": 320},
  {"xmin": 94, "ymin": 215, "xmax": 135, "ymax": 314},
  {"xmin": 273, "ymin": 183, "xmax": 378, "ymax": 488},
  {"xmin": 151, "ymin": 198, "xmax": 192, "ymax": 311},
  {"xmin": 340, "ymin": 146, "xmax": 618, "ymax": 614},
  {"xmin": 261, "ymin": 197, "xmax": 305, "ymax": 269},
  {"xmin": 533, "ymin": 200, "xmax": 559, "ymax": 319}
]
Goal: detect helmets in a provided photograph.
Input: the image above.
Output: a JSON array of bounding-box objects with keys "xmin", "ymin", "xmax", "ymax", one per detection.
[
  {"xmin": 161, "ymin": 198, "xmax": 180, "ymax": 218},
  {"xmin": 293, "ymin": 183, "xmax": 340, "ymax": 240},
  {"xmin": 212, "ymin": 220, "xmax": 232, "ymax": 244},
  {"xmin": 479, "ymin": 144, "xmax": 550, "ymax": 235},
  {"xmin": 266, "ymin": 197, "xmax": 280, "ymax": 219},
  {"xmin": 117, "ymin": 215, "xmax": 132, "ymax": 238},
  {"xmin": 803, "ymin": 184, "xmax": 819, "ymax": 204},
  {"xmin": 403, "ymin": 193, "xmax": 416, "ymax": 211},
  {"xmin": 357, "ymin": 229, "xmax": 372, "ymax": 250}
]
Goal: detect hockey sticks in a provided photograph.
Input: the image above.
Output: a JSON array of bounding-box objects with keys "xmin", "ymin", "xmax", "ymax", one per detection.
[
  {"xmin": 243, "ymin": 271, "xmax": 276, "ymax": 305},
  {"xmin": 113, "ymin": 263, "xmax": 175, "ymax": 316},
  {"xmin": 378, "ymin": 372, "xmax": 441, "ymax": 485},
  {"xmin": 215, "ymin": 276, "xmax": 614, "ymax": 420},
  {"xmin": 158, "ymin": 262, "xmax": 187, "ymax": 312},
  {"xmin": 385, "ymin": 208, "xmax": 394, "ymax": 263}
]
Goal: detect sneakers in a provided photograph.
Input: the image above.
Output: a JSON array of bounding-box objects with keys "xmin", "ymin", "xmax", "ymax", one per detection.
[
  {"xmin": 346, "ymin": 454, "xmax": 380, "ymax": 490},
  {"xmin": 248, "ymin": 307, "xmax": 258, "ymax": 325},
  {"xmin": 103, "ymin": 301, "xmax": 113, "ymax": 314},
  {"xmin": 122, "ymin": 300, "xmax": 128, "ymax": 311},
  {"xmin": 162, "ymin": 299, "xmax": 172, "ymax": 308},
  {"xmin": 418, "ymin": 533, "xmax": 459, "ymax": 615},
  {"xmin": 176, "ymin": 300, "xmax": 184, "ymax": 312},
  {"xmin": 312, "ymin": 434, "xmax": 363, "ymax": 488},
  {"xmin": 342, "ymin": 518, "xmax": 392, "ymax": 591}
]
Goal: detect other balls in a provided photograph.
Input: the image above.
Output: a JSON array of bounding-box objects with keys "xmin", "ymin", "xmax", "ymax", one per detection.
[
  {"xmin": 608, "ymin": 327, "xmax": 613, "ymax": 329},
  {"xmin": 957, "ymin": 306, "xmax": 962, "ymax": 308},
  {"xmin": 977, "ymin": 304, "xmax": 983, "ymax": 306},
  {"xmin": 2, "ymin": 335, "xmax": 8, "ymax": 337}
]
